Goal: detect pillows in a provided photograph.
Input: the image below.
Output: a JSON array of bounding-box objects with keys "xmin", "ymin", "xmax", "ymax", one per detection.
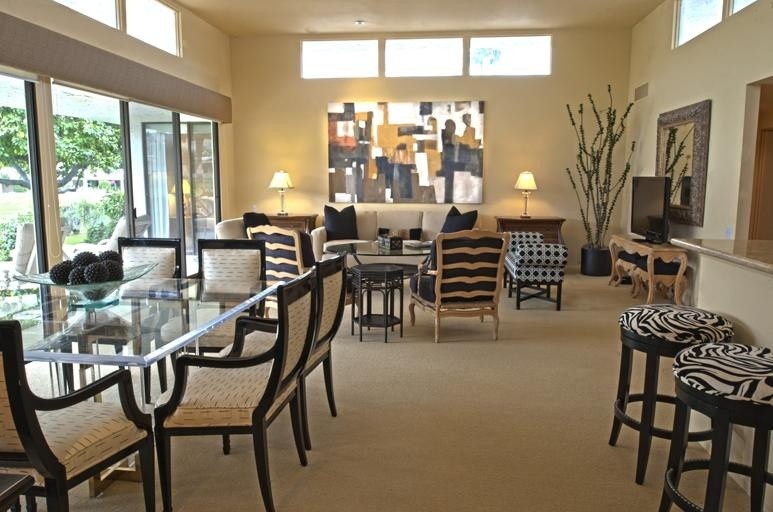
[
  {"xmin": 299, "ymin": 231, "xmax": 314, "ymax": 264},
  {"xmin": 323, "ymin": 205, "xmax": 358, "ymax": 240},
  {"xmin": 440, "ymin": 207, "xmax": 478, "ymax": 231},
  {"xmin": 243, "ymin": 211, "xmax": 272, "ymax": 230}
]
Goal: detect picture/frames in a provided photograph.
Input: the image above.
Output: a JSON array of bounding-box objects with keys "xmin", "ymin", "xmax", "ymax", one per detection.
[{"xmin": 655, "ymin": 98, "xmax": 711, "ymax": 226}]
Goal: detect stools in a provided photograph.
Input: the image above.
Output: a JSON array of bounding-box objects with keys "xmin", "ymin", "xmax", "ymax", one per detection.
[
  {"xmin": 659, "ymin": 342, "xmax": 773, "ymax": 512},
  {"xmin": 633, "ymin": 262, "xmax": 689, "ymax": 302},
  {"xmin": 609, "ymin": 303, "xmax": 736, "ymax": 485},
  {"xmin": 613, "ymin": 251, "xmax": 636, "ymax": 291}
]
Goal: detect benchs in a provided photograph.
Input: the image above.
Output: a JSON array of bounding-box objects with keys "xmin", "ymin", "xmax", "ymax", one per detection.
[{"xmin": 504, "ymin": 231, "xmax": 569, "ymax": 311}]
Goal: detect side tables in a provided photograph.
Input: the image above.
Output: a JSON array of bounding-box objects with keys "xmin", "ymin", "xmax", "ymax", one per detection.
[{"xmin": 350, "ymin": 264, "xmax": 404, "ymax": 344}]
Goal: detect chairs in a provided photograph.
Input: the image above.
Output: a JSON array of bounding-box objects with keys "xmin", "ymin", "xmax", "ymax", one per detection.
[
  {"xmin": 13, "ymin": 220, "xmax": 71, "ymax": 280},
  {"xmin": 1, "ymin": 319, "xmax": 155, "ymax": 511},
  {"xmin": 87, "ymin": 237, "xmax": 181, "ymax": 404},
  {"xmin": 76, "ymin": 215, "xmax": 151, "ymax": 252},
  {"xmin": 154, "ymin": 265, "xmax": 317, "ymax": 511},
  {"xmin": 219, "ymin": 249, "xmax": 349, "ymax": 449},
  {"xmin": 169, "ymin": 240, "xmax": 266, "ymax": 378},
  {"xmin": 408, "ymin": 230, "xmax": 506, "ymax": 343}
]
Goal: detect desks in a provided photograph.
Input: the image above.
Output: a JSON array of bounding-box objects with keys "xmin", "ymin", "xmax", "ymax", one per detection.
[
  {"xmin": 607, "ymin": 234, "xmax": 688, "ymax": 307},
  {"xmin": 327, "ymin": 243, "xmax": 432, "ymax": 266},
  {"xmin": 23, "ymin": 276, "xmax": 284, "ymax": 495}
]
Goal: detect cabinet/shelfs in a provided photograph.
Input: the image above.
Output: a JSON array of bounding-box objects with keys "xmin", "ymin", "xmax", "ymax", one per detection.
[
  {"xmin": 495, "ymin": 216, "xmax": 566, "ymax": 245},
  {"xmin": 268, "ymin": 213, "xmax": 319, "ymax": 234}
]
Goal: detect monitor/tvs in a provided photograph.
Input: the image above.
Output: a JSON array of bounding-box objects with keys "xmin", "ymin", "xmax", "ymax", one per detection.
[{"xmin": 629, "ymin": 175, "xmax": 672, "ymax": 246}]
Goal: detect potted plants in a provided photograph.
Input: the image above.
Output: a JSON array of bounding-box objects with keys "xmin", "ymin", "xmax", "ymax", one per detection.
[{"xmin": 565, "ymin": 84, "xmax": 636, "ymax": 276}]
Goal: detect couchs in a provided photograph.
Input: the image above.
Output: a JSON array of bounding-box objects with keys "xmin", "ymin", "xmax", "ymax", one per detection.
[
  {"xmin": 312, "ymin": 210, "xmax": 482, "ymax": 263},
  {"xmin": 214, "ymin": 216, "xmax": 246, "ymax": 241}
]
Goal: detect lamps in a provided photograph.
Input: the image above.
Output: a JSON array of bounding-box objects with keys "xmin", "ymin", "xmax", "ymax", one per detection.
[
  {"xmin": 515, "ymin": 171, "xmax": 538, "ymax": 219},
  {"xmin": 268, "ymin": 170, "xmax": 295, "ymax": 216},
  {"xmin": 172, "ymin": 179, "xmax": 191, "ymax": 216}
]
